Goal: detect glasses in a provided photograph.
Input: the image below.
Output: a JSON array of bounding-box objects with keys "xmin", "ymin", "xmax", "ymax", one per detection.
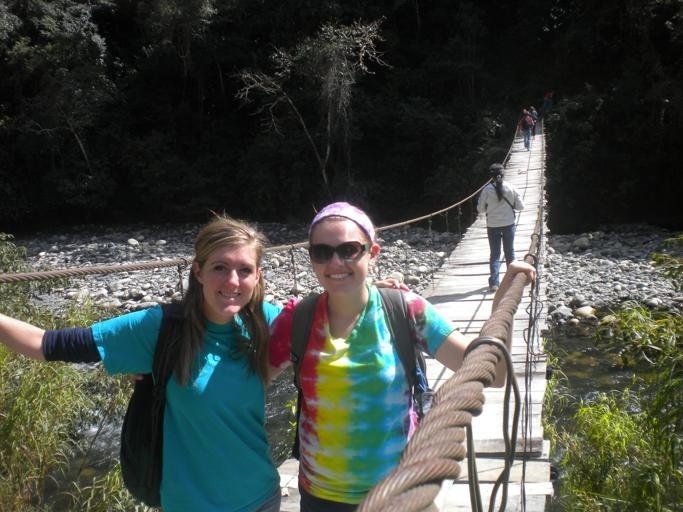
[{"xmin": 310, "ymin": 242, "xmax": 369, "ymax": 265}]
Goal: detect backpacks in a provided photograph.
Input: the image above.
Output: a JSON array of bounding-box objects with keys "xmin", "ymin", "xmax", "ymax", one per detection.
[
  {"xmin": 120, "ymin": 305, "xmax": 184, "ymax": 508},
  {"xmin": 290, "ymin": 287, "xmax": 431, "ymax": 460}
]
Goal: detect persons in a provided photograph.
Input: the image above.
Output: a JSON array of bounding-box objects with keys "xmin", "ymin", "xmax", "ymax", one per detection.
[
  {"xmin": 0, "ymin": 213, "xmax": 410, "ymax": 512},
  {"xmin": 477, "ymin": 164, "xmax": 524, "ymax": 291},
  {"xmin": 267, "ymin": 202, "xmax": 536, "ymax": 512},
  {"xmin": 518, "ymin": 105, "xmax": 539, "ymax": 151}
]
